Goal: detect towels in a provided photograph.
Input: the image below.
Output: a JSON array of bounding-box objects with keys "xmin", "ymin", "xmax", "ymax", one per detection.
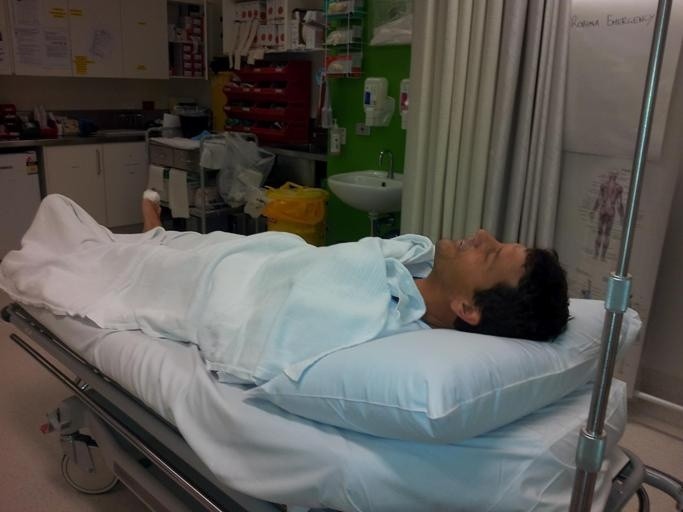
[
  {"xmin": 166, "ymin": 167, "xmax": 191, "ymax": 220},
  {"xmin": 146, "ymin": 163, "xmax": 165, "ymax": 194}
]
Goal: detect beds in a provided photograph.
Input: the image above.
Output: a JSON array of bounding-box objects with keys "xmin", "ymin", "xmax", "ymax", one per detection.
[{"xmin": 0, "ymin": 273, "xmax": 681, "ymax": 512}]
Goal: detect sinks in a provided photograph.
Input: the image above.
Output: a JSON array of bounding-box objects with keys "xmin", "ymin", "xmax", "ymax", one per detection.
[
  {"xmin": 327, "ymin": 169, "xmax": 403, "ymax": 214},
  {"xmin": 100, "ymin": 129, "xmax": 145, "ymax": 136}
]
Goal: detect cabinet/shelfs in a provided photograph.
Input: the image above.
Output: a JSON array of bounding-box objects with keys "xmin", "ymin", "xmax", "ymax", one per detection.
[
  {"xmin": 121, "ymin": 0, "xmax": 212, "ymax": 81},
  {"xmin": 219, "ymin": 0, "xmax": 325, "ymax": 54},
  {"xmin": 219, "ymin": 61, "xmax": 308, "ymax": 145},
  {"xmin": 9, "ymin": 1, "xmax": 121, "ymax": 81},
  {"xmin": 142, "ymin": 123, "xmax": 255, "ymax": 235},
  {"xmin": 39, "ymin": 143, "xmax": 107, "ymax": 229},
  {"xmin": 101, "ymin": 141, "xmax": 148, "ymax": 229},
  {"xmin": 0, "ymin": 1, "xmax": 14, "ymax": 77},
  {"xmin": 324, "ymin": 0, "xmax": 367, "ymax": 81}
]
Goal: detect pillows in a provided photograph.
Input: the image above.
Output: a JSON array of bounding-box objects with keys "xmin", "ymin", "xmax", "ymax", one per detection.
[{"xmin": 235, "ymin": 291, "xmax": 645, "ymax": 448}]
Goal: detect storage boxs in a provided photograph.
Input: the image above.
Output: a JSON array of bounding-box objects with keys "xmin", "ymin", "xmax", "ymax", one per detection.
[
  {"xmin": 234, "ymin": 2, "xmax": 286, "ymax": 51},
  {"xmin": 152, "ymin": 164, "xmax": 199, "ymax": 207}
]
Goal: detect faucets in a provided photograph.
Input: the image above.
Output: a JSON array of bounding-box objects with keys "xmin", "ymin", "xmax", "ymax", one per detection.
[
  {"xmin": 378, "ymin": 149, "xmax": 395, "ymax": 180},
  {"xmin": 119, "ymin": 111, "xmax": 143, "ymax": 122}
]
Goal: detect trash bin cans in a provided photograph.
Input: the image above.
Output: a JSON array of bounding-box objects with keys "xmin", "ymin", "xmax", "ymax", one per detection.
[{"xmin": 260, "ymin": 186, "xmax": 329, "ymax": 246}]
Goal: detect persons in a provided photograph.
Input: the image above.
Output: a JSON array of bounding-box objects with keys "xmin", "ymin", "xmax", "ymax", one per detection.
[{"xmin": 42, "ymin": 188, "xmax": 569, "ymax": 384}]
